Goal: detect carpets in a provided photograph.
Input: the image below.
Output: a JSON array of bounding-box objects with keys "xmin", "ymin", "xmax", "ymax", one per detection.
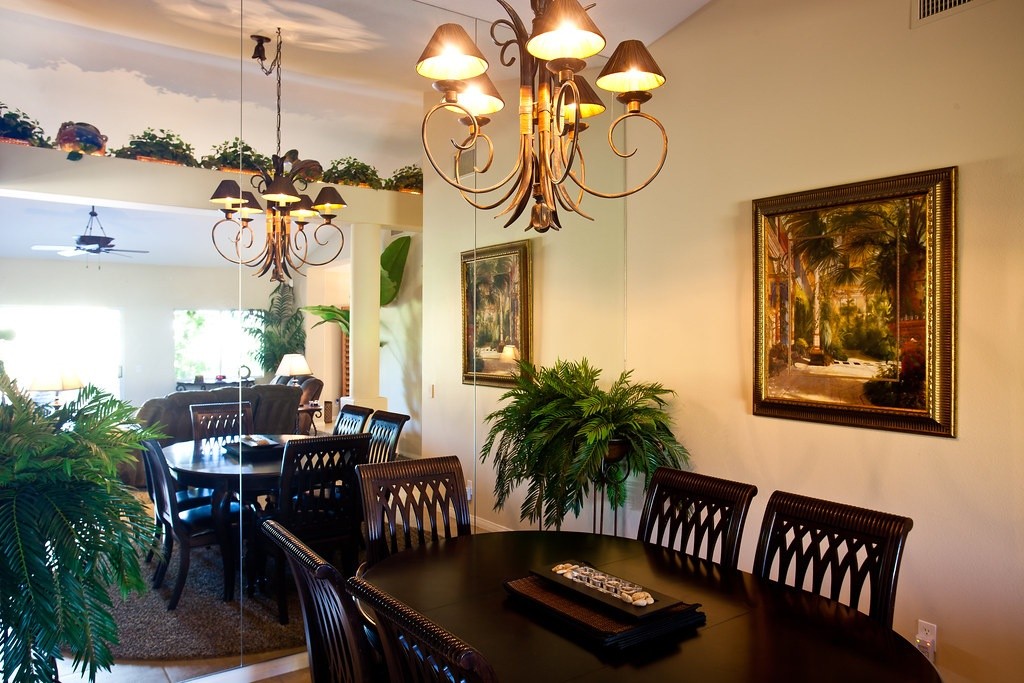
[{"xmin": 11, "ymin": 522, "xmax": 445, "ymax": 659}]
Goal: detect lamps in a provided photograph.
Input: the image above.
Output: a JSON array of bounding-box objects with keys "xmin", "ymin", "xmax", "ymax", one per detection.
[
  {"xmin": 30, "ymin": 376, "xmax": 84, "ymax": 419},
  {"xmin": 416, "ymin": 0, "xmax": 668, "ymax": 232},
  {"xmin": 276, "ymin": 353, "xmax": 314, "ymax": 407},
  {"xmin": 211, "ymin": 26, "xmax": 348, "ymax": 286}
]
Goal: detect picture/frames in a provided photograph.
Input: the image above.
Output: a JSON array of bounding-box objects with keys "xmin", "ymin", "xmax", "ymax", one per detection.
[
  {"xmin": 750, "ymin": 164, "xmax": 960, "ymax": 438},
  {"xmin": 458, "ymin": 238, "xmax": 534, "ymax": 388}
]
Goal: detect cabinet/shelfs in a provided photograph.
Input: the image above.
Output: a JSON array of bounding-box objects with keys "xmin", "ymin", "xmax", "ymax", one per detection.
[{"xmin": 341, "ymin": 306, "xmax": 350, "ymax": 397}]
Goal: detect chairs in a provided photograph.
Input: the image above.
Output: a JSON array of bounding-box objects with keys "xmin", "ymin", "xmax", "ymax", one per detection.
[
  {"xmin": 137, "ymin": 400, "xmax": 500, "ymax": 683},
  {"xmin": 752, "ymin": 489, "xmax": 914, "ymax": 629},
  {"xmin": 637, "ymin": 468, "xmax": 758, "ymax": 570}
]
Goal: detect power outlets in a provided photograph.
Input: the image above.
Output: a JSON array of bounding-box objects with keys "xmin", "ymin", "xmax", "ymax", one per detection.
[
  {"xmin": 466, "ymin": 479, "xmax": 472, "ymax": 495},
  {"xmin": 918, "ymin": 620, "xmax": 937, "ymax": 652}
]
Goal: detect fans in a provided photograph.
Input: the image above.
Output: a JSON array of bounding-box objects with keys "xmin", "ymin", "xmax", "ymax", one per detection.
[{"xmin": 32, "ymin": 205, "xmax": 150, "ymax": 258}]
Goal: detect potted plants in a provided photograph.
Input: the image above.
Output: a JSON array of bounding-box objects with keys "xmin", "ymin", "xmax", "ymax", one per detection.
[
  {"xmin": 478, "ymin": 355, "xmax": 692, "ymax": 532},
  {"xmin": 0, "ymin": 102, "xmax": 423, "ymax": 196}
]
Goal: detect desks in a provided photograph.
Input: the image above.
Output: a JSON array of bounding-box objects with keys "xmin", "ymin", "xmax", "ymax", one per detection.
[
  {"xmin": 160, "ymin": 434, "xmax": 338, "ymax": 602},
  {"xmin": 176, "ymin": 377, "xmax": 255, "ymax": 391},
  {"xmin": 361, "ymin": 531, "xmax": 942, "ymax": 683}
]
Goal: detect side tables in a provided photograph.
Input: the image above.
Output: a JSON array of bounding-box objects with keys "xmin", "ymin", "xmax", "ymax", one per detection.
[{"xmin": 293, "ymin": 406, "xmax": 322, "ymax": 435}]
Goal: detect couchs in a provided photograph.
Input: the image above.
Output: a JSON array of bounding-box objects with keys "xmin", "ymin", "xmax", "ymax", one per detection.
[
  {"xmin": 109, "ymin": 385, "xmax": 304, "ymax": 488},
  {"xmin": 269, "ymin": 376, "xmax": 325, "ymax": 434}
]
